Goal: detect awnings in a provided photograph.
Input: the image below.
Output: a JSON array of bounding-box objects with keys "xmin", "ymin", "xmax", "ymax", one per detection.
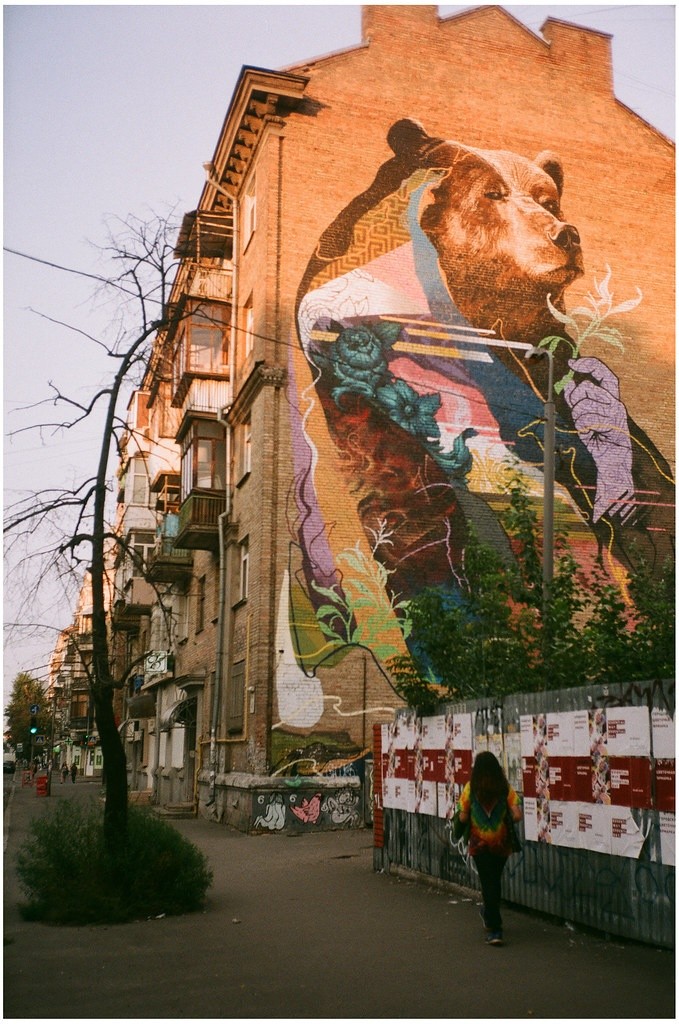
[
  {"xmin": 53, "ymin": 745, "xmax": 61, "ymax": 753},
  {"xmin": 117, "ymin": 720, "xmax": 135, "ymax": 738},
  {"xmin": 158, "ymin": 695, "xmax": 198, "ymax": 728}
]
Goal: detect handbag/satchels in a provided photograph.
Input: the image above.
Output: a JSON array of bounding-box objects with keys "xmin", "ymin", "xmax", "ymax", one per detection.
[{"xmin": 504, "ymin": 797, "xmax": 522, "ymax": 854}]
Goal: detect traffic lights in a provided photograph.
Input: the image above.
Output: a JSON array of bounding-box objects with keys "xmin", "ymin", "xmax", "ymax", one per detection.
[{"xmin": 30, "ymin": 717, "xmax": 37, "ymax": 734}]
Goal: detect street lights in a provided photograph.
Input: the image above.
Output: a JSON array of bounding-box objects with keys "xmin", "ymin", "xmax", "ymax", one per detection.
[{"xmin": 523, "ymin": 345, "xmax": 556, "ymax": 623}]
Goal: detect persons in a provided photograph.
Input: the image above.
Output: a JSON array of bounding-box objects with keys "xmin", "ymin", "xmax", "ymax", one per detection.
[
  {"xmin": 70, "ymin": 763, "xmax": 77, "ymax": 784},
  {"xmin": 60, "ymin": 761, "xmax": 70, "ymax": 784},
  {"xmin": 458, "ymin": 751, "xmax": 522, "ymax": 946}
]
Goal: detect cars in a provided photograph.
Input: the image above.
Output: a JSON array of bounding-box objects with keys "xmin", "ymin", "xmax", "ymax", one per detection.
[{"xmin": 3, "ymin": 760, "xmax": 15, "ymax": 773}]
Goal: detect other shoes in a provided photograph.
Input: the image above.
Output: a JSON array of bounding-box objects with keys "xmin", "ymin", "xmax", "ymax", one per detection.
[
  {"xmin": 72, "ymin": 782, "xmax": 75, "ymax": 784},
  {"xmin": 60, "ymin": 782, "xmax": 63, "ymax": 784}
]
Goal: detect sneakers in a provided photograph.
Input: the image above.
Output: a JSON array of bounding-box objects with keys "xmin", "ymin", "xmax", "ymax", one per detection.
[
  {"xmin": 484, "ymin": 928, "xmax": 504, "ymax": 946},
  {"xmin": 478, "ymin": 906, "xmax": 502, "ymax": 930}
]
